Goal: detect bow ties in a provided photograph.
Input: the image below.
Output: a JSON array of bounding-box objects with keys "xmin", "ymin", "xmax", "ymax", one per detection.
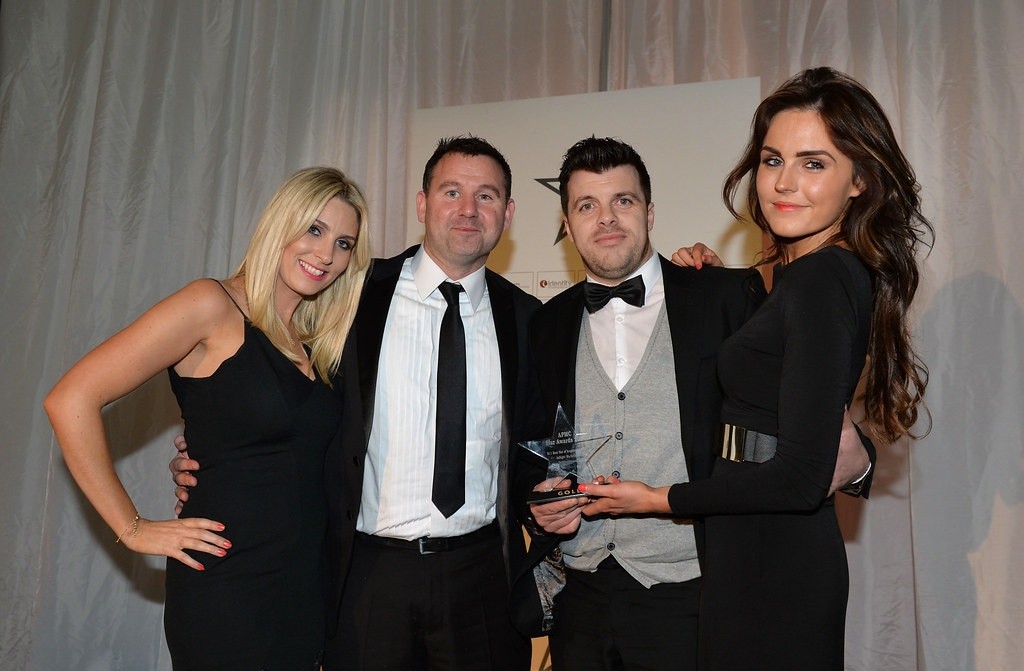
[{"xmin": 582, "ymin": 274, "xmax": 648, "ymax": 314}]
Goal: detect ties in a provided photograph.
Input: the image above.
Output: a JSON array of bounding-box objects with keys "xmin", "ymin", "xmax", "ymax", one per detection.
[{"xmin": 431, "ymin": 282, "xmax": 467, "ymax": 519}]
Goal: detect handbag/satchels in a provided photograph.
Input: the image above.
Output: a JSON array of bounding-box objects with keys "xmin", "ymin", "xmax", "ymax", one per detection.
[{"xmin": 825, "ymin": 405, "xmax": 873, "ymax": 499}]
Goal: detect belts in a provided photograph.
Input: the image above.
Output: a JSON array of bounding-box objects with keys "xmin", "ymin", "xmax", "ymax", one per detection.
[
  {"xmin": 720, "ymin": 426, "xmax": 779, "ymax": 463},
  {"xmin": 353, "ymin": 522, "xmax": 500, "ymax": 553}
]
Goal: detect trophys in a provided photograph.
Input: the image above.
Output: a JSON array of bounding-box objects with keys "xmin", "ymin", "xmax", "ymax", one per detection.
[{"xmin": 518, "ymin": 400, "xmax": 612, "ymax": 502}]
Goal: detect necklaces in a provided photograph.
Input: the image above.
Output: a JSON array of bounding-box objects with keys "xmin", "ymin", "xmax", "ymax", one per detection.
[{"xmin": 284, "ymin": 332, "xmax": 296, "ymax": 349}]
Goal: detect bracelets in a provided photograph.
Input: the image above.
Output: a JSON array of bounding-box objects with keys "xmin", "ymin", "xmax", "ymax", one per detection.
[
  {"xmin": 847, "ymin": 463, "xmax": 873, "ymax": 488},
  {"xmin": 115, "ymin": 512, "xmax": 143, "ymax": 542}
]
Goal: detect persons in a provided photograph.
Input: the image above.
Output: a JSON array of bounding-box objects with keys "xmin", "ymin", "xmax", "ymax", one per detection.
[
  {"xmin": 577, "ymin": 67, "xmax": 937, "ymax": 671},
  {"xmin": 528, "ymin": 132, "xmax": 877, "ymax": 671},
  {"xmin": 167, "ymin": 133, "xmax": 551, "ymax": 671},
  {"xmin": 44, "ymin": 168, "xmax": 375, "ymax": 671}
]
